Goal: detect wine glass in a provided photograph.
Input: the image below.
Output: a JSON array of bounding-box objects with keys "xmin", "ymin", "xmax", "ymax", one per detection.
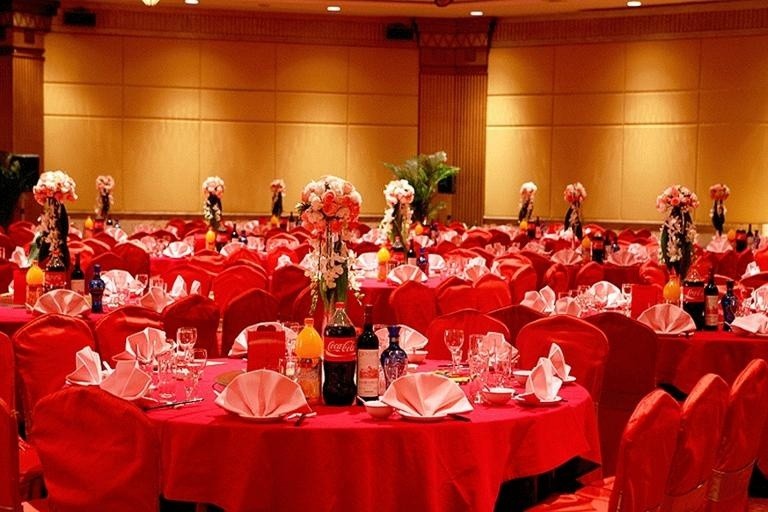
[
  {"xmin": 282, "ymin": 320, "xmax": 298, "ymax": 369},
  {"xmin": 555, "ymin": 285, "xmax": 634, "ymax": 317},
  {"xmin": 741, "ymin": 288, "xmax": 766, "ymax": 334},
  {"xmin": 156, "ymin": 328, "xmax": 210, "ymax": 405}
]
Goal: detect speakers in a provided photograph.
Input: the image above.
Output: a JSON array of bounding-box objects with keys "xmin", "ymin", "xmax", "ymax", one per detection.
[
  {"xmin": 11, "ymin": 154, "xmax": 39, "ymax": 192},
  {"xmin": 387, "ymin": 24, "xmax": 414, "ymax": 40},
  {"xmin": 63, "ymin": 12, "xmax": 96, "ymax": 27},
  {"xmin": 438, "ymin": 167, "xmax": 456, "ymax": 193}
]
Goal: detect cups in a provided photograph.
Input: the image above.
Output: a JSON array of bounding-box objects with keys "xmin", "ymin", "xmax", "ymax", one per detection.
[{"xmin": 443, "ymin": 329, "xmax": 513, "ymax": 406}]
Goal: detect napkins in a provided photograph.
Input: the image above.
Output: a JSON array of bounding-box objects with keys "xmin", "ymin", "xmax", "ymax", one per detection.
[
  {"xmin": 111, "ymin": 327, "xmax": 173, "ymax": 364},
  {"xmin": 380, "ymin": 372, "xmax": 477, "ymax": 417},
  {"xmin": 102, "ymin": 362, "xmax": 154, "ymax": 403},
  {"xmin": 372, "ymin": 325, "xmax": 430, "ymax": 354},
  {"xmin": 740, "ymin": 283, "xmax": 768, "ymax": 312},
  {"xmin": 729, "ymin": 313, "xmax": 768, "ymax": 337},
  {"xmin": 637, "ymin": 304, "xmax": 696, "ymax": 337},
  {"xmin": 215, "ymin": 369, "xmax": 313, "ymax": 417}
]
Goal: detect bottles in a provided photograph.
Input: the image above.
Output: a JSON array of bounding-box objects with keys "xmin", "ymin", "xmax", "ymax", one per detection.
[
  {"xmin": 6, "ymin": 212, "xmax": 189, "ymax": 313},
  {"xmin": 294, "ymin": 302, "xmax": 403, "ymax": 404},
  {"xmin": 198, "ymin": 213, "xmax": 768, "ymax": 280},
  {"xmin": 662, "ymin": 269, "xmax": 738, "ymax": 330}
]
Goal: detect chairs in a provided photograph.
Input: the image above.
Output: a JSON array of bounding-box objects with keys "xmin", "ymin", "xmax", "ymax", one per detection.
[
  {"xmin": 689, "ymin": 247, "xmax": 768, "ymax": 299},
  {"xmin": 526, "ymin": 388, "xmax": 680, "ymax": 512},
  {"xmin": 725, "ymin": 358, "xmax": 767, "ymax": 512},
  {"xmin": 488, "ymin": 304, "xmax": 546, "ymax": 340},
  {"xmin": 0, "ymin": 396, "xmax": 37, "ymax": 512},
  {"xmin": 422, "ymin": 309, "xmax": 512, "ymax": 361},
  {"xmin": 580, "ymin": 311, "xmax": 660, "ymax": 412},
  {"xmin": 512, "ymin": 314, "xmax": 610, "ymax": 396},
  {"xmin": 94, "ymin": 306, "xmax": 165, "ymax": 367},
  {"xmin": 353, "ymin": 222, "xmax": 656, "ymax": 325},
  {"xmin": 676, "ymin": 372, "xmax": 732, "ymax": 512},
  {"xmin": 0, "ymin": 316, "xmax": 99, "ymax": 435},
  {"xmin": 221, "ymin": 287, "xmax": 283, "ymax": 356},
  {"xmin": 0, "ymin": 217, "xmax": 320, "ymax": 333},
  {"xmin": 28, "ymin": 387, "xmax": 159, "ymax": 512},
  {"xmin": 161, "ymin": 295, "xmax": 220, "ymax": 358}
]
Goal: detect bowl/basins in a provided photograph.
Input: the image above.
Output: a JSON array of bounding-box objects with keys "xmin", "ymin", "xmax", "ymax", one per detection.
[
  {"xmin": 102, "ymin": 370, "xmax": 116, "ymax": 379},
  {"xmin": 406, "ymin": 351, "xmax": 428, "ymax": 364}
]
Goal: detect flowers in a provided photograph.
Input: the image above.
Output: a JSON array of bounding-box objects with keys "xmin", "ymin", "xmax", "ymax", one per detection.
[
  {"xmin": 563, "ymin": 179, "xmax": 588, "ymax": 232},
  {"xmin": 374, "ymin": 179, "xmax": 415, "ymax": 249},
  {"xmin": 707, "ymin": 181, "xmax": 731, "ymax": 236},
  {"xmin": 269, "ymin": 176, "xmax": 286, "ymax": 218},
  {"xmin": 518, "ymin": 181, "xmax": 539, "ymax": 213},
  {"xmin": 31, "ymin": 167, "xmax": 80, "ymax": 271},
  {"xmin": 94, "ymin": 173, "xmax": 116, "ymax": 218},
  {"xmin": 293, "ymin": 174, "xmax": 366, "ymax": 313},
  {"xmin": 201, "ymin": 175, "xmax": 226, "ymax": 227},
  {"xmin": 654, "ymin": 179, "xmax": 703, "ymax": 266}
]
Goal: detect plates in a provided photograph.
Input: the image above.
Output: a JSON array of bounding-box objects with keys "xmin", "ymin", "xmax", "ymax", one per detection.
[
  {"xmin": 400, "ymin": 411, "xmax": 447, "ymax": 422},
  {"xmin": 515, "ymin": 370, "xmax": 580, "ymax": 406},
  {"xmin": 236, "ymin": 412, "xmax": 287, "ymax": 423}
]
